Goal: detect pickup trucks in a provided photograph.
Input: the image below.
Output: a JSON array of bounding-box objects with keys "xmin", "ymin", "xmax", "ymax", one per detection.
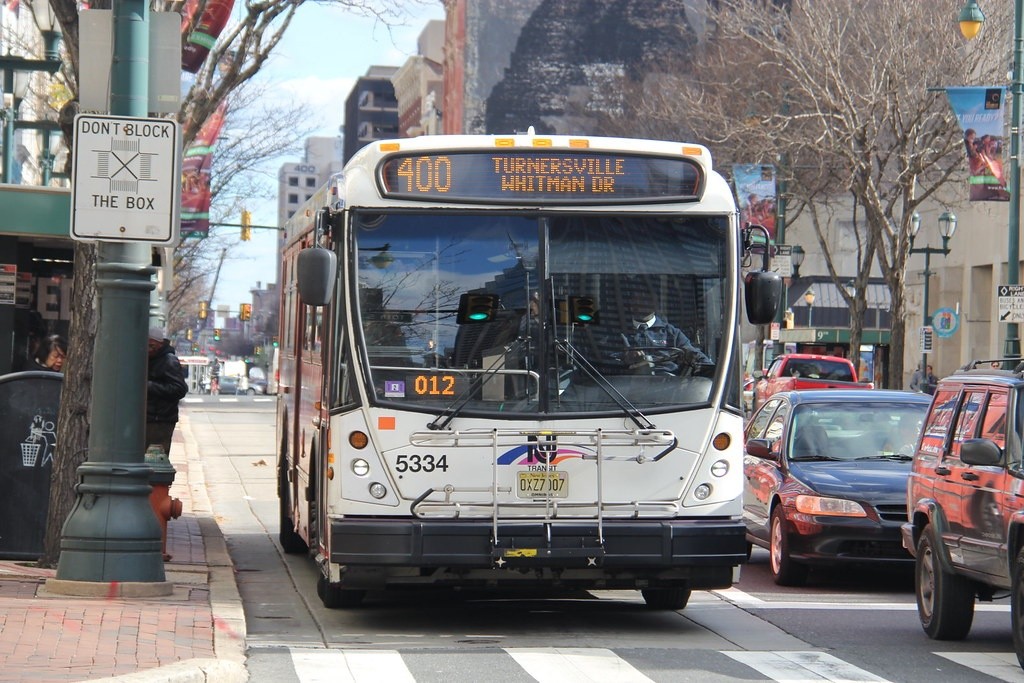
[{"xmin": 742, "ymin": 353, "xmax": 877, "ymax": 415}]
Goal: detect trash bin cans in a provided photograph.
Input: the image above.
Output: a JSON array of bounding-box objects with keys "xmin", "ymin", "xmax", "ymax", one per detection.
[{"xmin": 0, "ymin": 371, "xmax": 69, "ymax": 558}]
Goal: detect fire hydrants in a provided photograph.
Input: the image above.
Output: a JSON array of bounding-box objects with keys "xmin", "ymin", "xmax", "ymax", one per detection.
[{"xmin": 146, "ymin": 443, "xmax": 184, "ymax": 562}]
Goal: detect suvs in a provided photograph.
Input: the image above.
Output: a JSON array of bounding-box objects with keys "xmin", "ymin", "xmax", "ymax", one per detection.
[{"xmin": 900, "ymin": 357, "xmax": 1024, "ymax": 669}]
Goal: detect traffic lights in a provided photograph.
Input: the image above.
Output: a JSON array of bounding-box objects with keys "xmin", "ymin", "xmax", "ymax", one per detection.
[
  {"xmin": 213, "ymin": 329, "xmax": 220, "ymax": 341},
  {"xmin": 273, "ymin": 337, "xmax": 278, "ymax": 347},
  {"xmin": 245, "ymin": 358, "xmax": 250, "ymax": 363},
  {"xmin": 192, "ymin": 343, "xmax": 197, "ymax": 351},
  {"xmin": 241, "ymin": 304, "xmax": 252, "ymax": 320}
]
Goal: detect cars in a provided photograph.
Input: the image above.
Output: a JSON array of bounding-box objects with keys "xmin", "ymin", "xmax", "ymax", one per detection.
[
  {"xmin": 742, "ymin": 389, "xmax": 936, "ymax": 589},
  {"xmin": 199, "ymin": 374, "xmax": 264, "ymax": 395}
]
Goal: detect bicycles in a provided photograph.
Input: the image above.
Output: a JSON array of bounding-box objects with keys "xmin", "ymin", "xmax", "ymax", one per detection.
[{"xmin": 210, "ymin": 375, "xmax": 221, "ymax": 395}]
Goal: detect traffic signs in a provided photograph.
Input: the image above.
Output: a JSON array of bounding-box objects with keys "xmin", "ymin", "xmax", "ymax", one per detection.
[{"xmin": 997, "ymin": 286, "xmax": 1024, "ymax": 322}]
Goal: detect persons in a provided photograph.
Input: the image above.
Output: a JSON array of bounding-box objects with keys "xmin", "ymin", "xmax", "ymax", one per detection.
[
  {"xmin": 910, "ymin": 359, "xmax": 930, "ymax": 393},
  {"xmin": 991, "ymin": 362, "xmax": 1000, "ymax": 369},
  {"xmin": 210, "ymin": 378, "xmax": 220, "ymax": 396},
  {"xmin": 964, "ymin": 128, "xmax": 1009, "ymax": 200},
  {"xmin": 882, "ymin": 411, "xmax": 923, "ymax": 457},
  {"xmin": 926, "ymin": 365, "xmax": 939, "ymax": 395},
  {"xmin": 741, "ymin": 193, "xmax": 775, "ymax": 240},
  {"xmin": 211, "ymin": 357, "xmax": 221, "ymax": 384},
  {"xmin": 26, "ymin": 335, "xmax": 68, "ymax": 372},
  {"xmin": 197, "ymin": 375, "xmax": 206, "ymax": 395},
  {"xmin": 235, "ymin": 373, "xmax": 242, "ymax": 397},
  {"xmin": 598, "ymin": 279, "xmax": 714, "ymax": 376},
  {"xmin": 145, "ymin": 327, "xmax": 188, "ymax": 459}
]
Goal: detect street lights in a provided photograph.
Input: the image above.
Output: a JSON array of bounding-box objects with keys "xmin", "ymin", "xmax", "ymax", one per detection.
[
  {"xmin": 905, "ymin": 208, "xmax": 958, "ymax": 392},
  {"xmin": 804, "ymin": 289, "xmax": 816, "ymax": 330},
  {"xmin": 960, "ymin": 0, "xmax": 1024, "ymax": 372}
]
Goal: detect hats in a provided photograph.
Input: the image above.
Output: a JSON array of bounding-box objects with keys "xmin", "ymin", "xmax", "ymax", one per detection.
[{"xmin": 149, "ymin": 325, "xmax": 164, "ymax": 342}]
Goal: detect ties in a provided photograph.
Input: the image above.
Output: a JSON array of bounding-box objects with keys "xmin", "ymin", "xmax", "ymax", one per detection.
[{"xmin": 640, "ymin": 324, "xmax": 648, "ymax": 331}]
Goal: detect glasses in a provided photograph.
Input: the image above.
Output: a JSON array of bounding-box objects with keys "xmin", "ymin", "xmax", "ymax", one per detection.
[
  {"xmin": 149, "ymin": 343, "xmax": 160, "ymax": 349},
  {"xmin": 992, "ymin": 365, "xmax": 999, "ymax": 367}
]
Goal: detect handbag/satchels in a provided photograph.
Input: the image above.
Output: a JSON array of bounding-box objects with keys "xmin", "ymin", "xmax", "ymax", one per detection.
[{"xmin": 910, "ymin": 370, "xmax": 918, "ymax": 389}]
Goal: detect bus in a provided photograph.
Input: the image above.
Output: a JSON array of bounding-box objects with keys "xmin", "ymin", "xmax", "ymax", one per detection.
[{"xmin": 277, "ymin": 135, "xmax": 781, "ymax": 614}]
